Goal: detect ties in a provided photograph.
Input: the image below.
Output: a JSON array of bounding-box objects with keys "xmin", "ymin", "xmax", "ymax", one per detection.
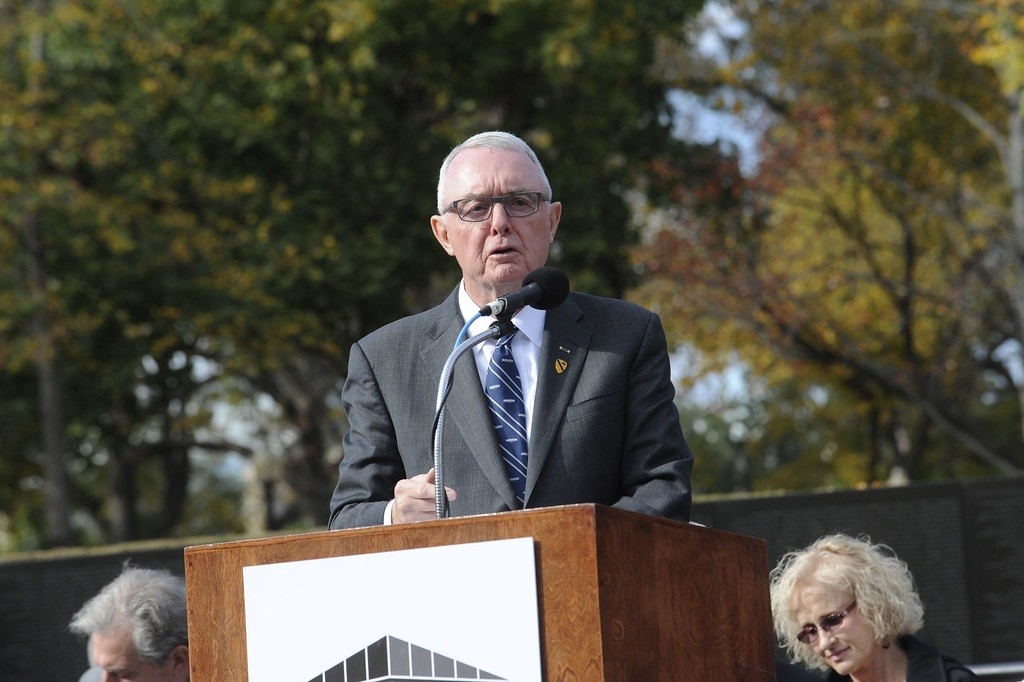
[{"xmin": 482, "ymin": 326, "xmax": 527, "ymax": 510}]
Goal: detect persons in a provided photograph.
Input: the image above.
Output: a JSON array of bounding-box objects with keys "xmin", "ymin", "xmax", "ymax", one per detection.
[
  {"xmin": 69, "ymin": 569, "xmax": 190, "ymax": 682},
  {"xmin": 327, "ymin": 132, "xmax": 694, "ymax": 530},
  {"xmin": 769, "ymin": 534, "xmax": 980, "ymax": 682}
]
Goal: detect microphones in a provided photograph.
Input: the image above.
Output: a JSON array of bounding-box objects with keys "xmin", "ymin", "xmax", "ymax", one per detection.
[{"xmin": 480, "ymin": 267, "xmax": 570, "ymax": 317}]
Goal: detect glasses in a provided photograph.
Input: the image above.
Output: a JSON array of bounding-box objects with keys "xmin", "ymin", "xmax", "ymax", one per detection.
[
  {"xmin": 441, "ymin": 191, "xmax": 551, "ymax": 223},
  {"xmin": 797, "ymin": 599, "xmax": 858, "ymax": 644}
]
